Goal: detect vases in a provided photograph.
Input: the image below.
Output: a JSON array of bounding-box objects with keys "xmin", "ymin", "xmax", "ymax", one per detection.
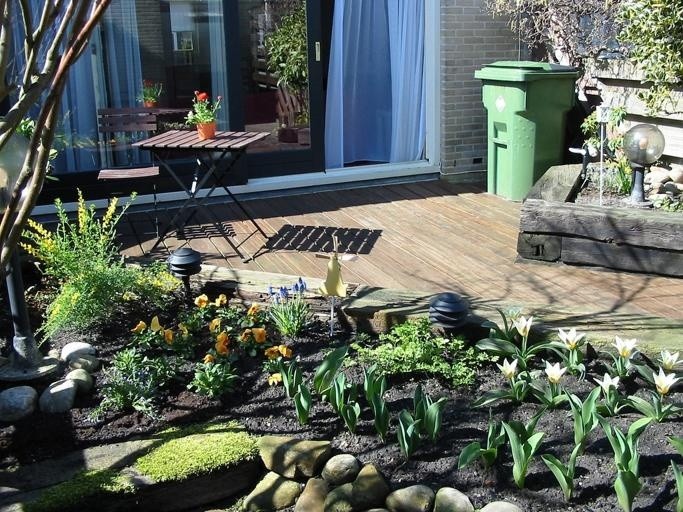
[
  {"xmin": 144, "ymin": 98, "xmax": 156, "ymax": 107},
  {"xmin": 194, "ymin": 121, "xmax": 218, "ymax": 139}
]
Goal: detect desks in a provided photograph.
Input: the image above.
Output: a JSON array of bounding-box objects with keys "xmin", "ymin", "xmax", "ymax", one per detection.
[{"xmin": 132, "ymin": 128, "xmax": 272, "ymax": 261}]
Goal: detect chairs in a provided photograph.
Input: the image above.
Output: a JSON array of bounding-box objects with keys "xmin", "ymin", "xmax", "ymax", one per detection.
[{"xmin": 93, "ymin": 108, "xmax": 165, "ymax": 237}]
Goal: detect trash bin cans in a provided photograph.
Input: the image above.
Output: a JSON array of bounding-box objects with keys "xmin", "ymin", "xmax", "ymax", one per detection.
[{"xmin": 474, "ymin": 61, "xmax": 581, "ymax": 202}]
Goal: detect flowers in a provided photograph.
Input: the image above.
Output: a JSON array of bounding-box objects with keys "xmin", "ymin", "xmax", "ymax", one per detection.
[
  {"xmin": 135, "ymin": 78, "xmax": 164, "ymax": 104},
  {"xmin": 181, "ymin": 90, "xmax": 224, "ymax": 123}
]
